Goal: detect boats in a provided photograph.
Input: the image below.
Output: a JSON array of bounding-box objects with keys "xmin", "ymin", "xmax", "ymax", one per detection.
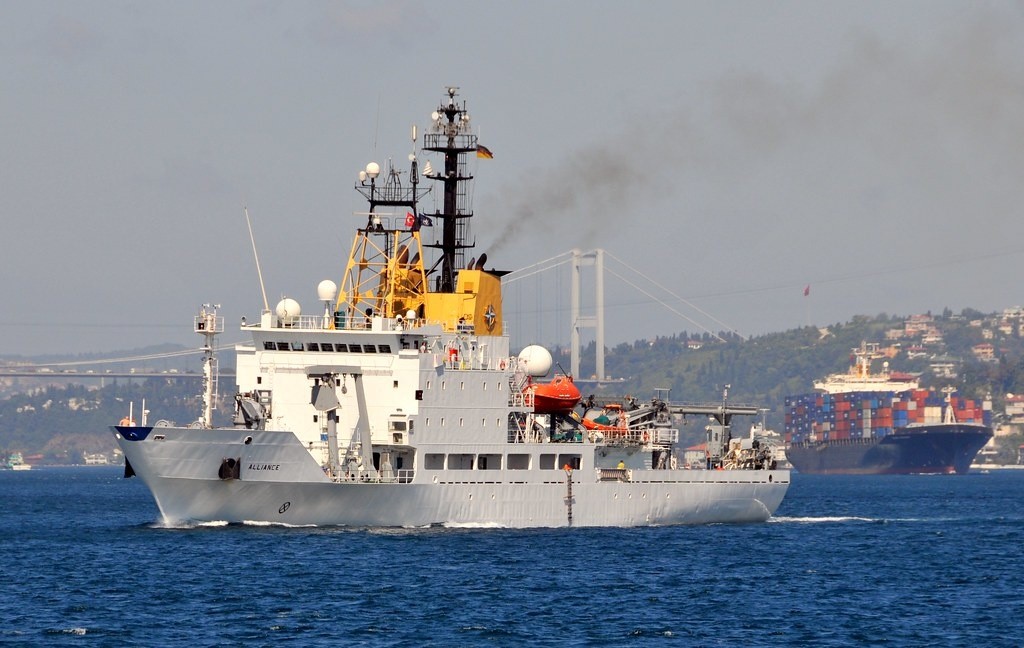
[
  {"xmin": 520, "ymin": 374, "xmax": 582, "ymax": 413},
  {"xmin": 12, "ymin": 464, "xmax": 31, "ymax": 471}
]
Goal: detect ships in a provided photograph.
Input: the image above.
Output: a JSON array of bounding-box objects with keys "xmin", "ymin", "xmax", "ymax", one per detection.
[
  {"xmin": 783, "ymin": 340, "xmax": 995, "ymax": 475},
  {"xmin": 108, "ymin": 87, "xmax": 794, "ymax": 530}
]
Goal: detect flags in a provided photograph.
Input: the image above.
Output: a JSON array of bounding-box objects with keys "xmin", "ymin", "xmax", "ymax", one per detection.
[
  {"xmin": 405, "ymin": 212, "xmax": 433, "ymax": 227},
  {"xmin": 477, "ymin": 143, "xmax": 493, "ymax": 159}
]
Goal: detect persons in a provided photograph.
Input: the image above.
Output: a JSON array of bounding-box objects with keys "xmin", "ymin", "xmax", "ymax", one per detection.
[
  {"xmin": 564, "ymin": 459, "xmax": 574, "ymax": 470},
  {"xmin": 704, "ymin": 450, "xmax": 710, "ymax": 468},
  {"xmin": 617, "ymin": 460, "xmax": 625, "ymax": 469},
  {"xmin": 119, "ymin": 416, "xmax": 136, "ymax": 426}
]
